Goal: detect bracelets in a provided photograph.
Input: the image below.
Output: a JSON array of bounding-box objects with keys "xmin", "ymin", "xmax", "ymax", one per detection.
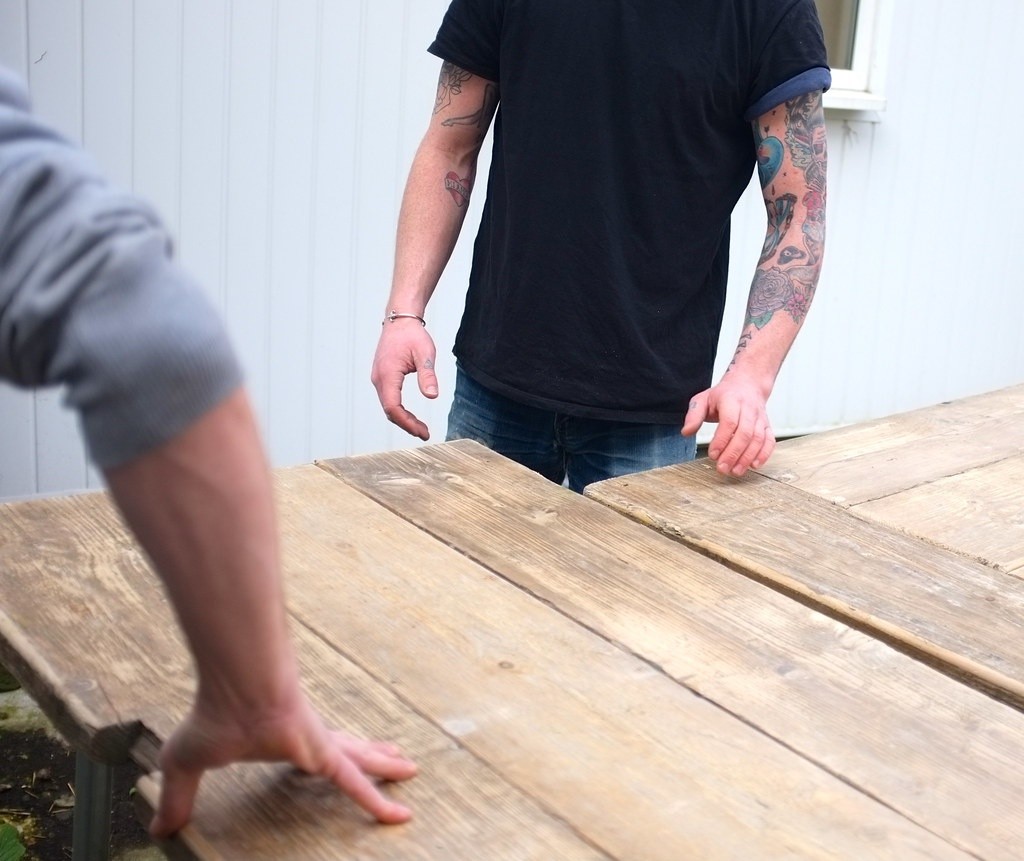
[{"xmin": 381, "ymin": 310, "xmax": 427, "ymax": 328}]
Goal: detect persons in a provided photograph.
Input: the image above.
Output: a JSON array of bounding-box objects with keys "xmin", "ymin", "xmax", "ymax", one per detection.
[
  {"xmin": 370, "ymin": 0, "xmax": 831, "ymax": 494},
  {"xmin": 0, "ymin": 58, "xmax": 418, "ymax": 834}
]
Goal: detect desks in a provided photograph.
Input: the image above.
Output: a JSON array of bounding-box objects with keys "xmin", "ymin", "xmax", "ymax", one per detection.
[
  {"xmin": 583, "ymin": 378, "xmax": 1024, "ymax": 706},
  {"xmin": 0, "ymin": 438, "xmax": 1023, "ymax": 861}
]
